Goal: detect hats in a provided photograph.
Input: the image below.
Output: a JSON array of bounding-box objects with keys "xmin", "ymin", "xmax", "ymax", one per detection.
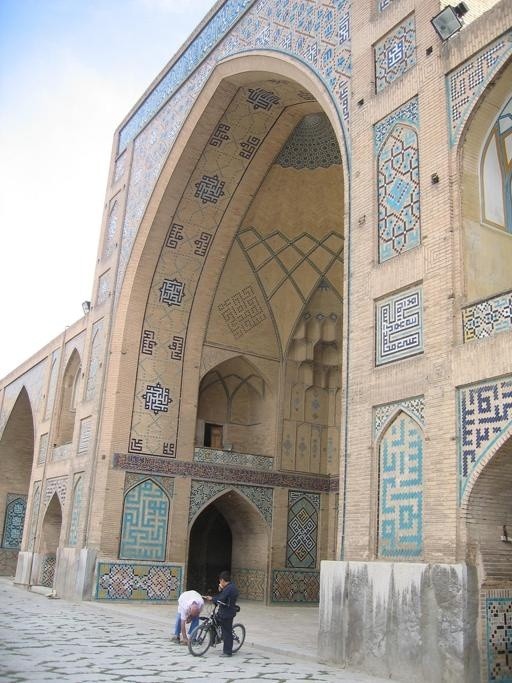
[{"xmin": 221, "ymin": 571, "xmax": 230, "ymax": 581}]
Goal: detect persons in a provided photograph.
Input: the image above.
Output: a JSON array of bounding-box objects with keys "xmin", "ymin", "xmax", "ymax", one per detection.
[
  {"xmin": 206, "ymin": 571, "xmax": 239, "ymax": 658},
  {"xmin": 170, "ymin": 590, "xmax": 204, "ymax": 646}
]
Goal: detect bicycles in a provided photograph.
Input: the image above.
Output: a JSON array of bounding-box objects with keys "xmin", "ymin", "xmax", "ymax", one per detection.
[{"xmin": 188, "ymin": 596, "xmax": 245, "ymax": 656}]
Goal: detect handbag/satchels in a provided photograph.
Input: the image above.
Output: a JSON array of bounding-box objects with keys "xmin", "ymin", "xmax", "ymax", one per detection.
[{"xmin": 218, "ymin": 608, "xmax": 235, "ymax": 620}]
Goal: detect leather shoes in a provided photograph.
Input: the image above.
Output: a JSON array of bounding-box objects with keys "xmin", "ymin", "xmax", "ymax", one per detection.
[{"xmin": 220, "ymin": 654, "xmax": 232, "ymax": 657}]
